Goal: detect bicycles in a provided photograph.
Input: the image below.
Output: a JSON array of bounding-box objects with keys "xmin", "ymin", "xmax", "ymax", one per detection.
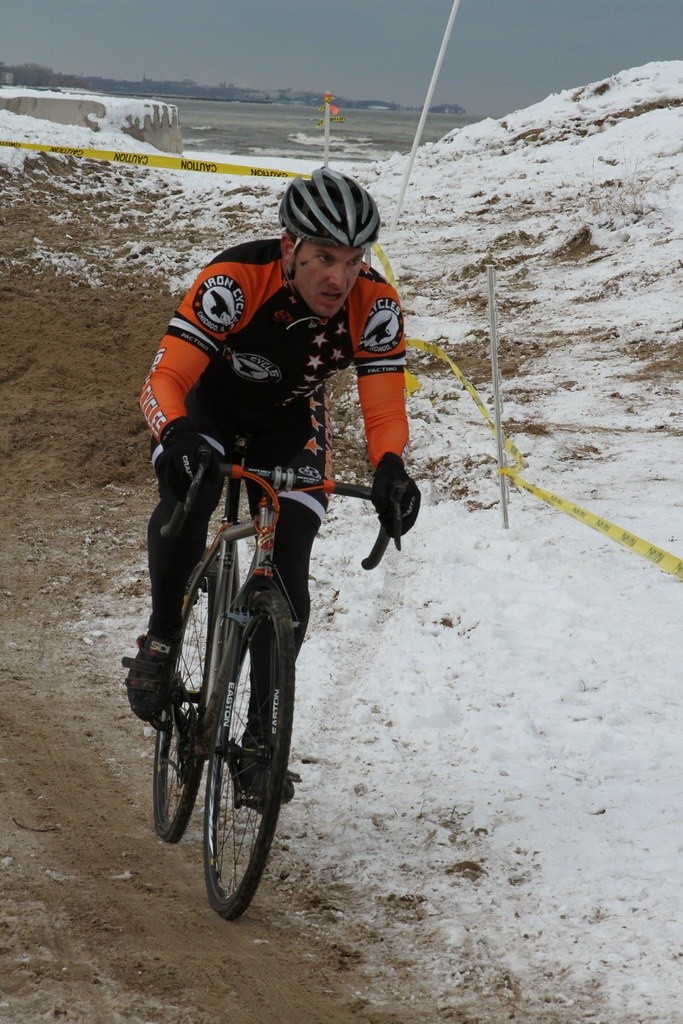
[{"xmin": 151, "ymin": 424, "xmax": 413, "ymax": 920}]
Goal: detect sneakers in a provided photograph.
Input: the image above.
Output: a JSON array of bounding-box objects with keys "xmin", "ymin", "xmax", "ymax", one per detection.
[
  {"xmin": 238, "ymin": 754, "xmax": 293, "ymax": 804},
  {"xmin": 127, "ymin": 613, "xmax": 181, "ymax": 722}
]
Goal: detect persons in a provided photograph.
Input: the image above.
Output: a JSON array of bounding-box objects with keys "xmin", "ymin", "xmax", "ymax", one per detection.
[{"xmin": 126, "ymin": 168, "xmax": 420, "ymax": 805}]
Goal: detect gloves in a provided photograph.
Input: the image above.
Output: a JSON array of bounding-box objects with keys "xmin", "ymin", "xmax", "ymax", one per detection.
[
  {"xmin": 160, "ymin": 419, "xmax": 205, "ymax": 482},
  {"xmin": 370, "ymin": 453, "xmax": 422, "ymax": 538}
]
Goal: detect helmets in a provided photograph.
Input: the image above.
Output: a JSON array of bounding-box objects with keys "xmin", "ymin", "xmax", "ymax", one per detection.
[{"xmin": 279, "ymin": 166, "xmax": 381, "ymax": 249}]
